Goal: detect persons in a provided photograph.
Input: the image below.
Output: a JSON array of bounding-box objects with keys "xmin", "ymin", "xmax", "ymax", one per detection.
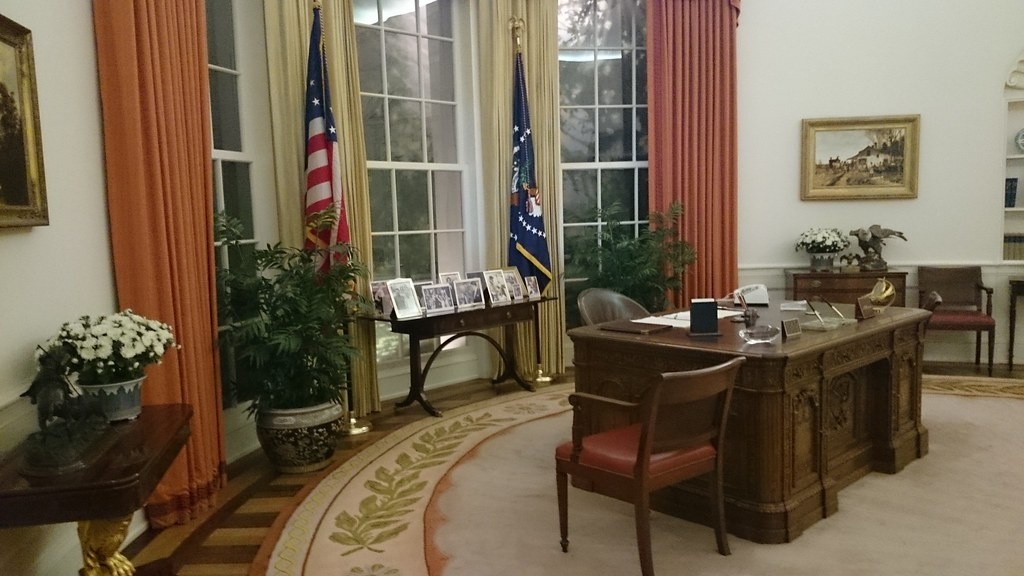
[
  {"xmin": 458, "ymin": 281, "xmax": 482, "ymax": 305},
  {"xmin": 528, "ymin": 281, "xmax": 537, "ymax": 294},
  {"xmin": 829, "ymin": 156, "xmax": 885, "ymax": 177},
  {"xmin": 508, "ymin": 276, "xmax": 521, "ymax": 297},
  {"xmin": 394, "ymin": 285, "xmax": 414, "ymax": 312},
  {"xmin": 447, "ymin": 277, "xmax": 456, "ymax": 306},
  {"xmin": 375, "ymin": 284, "xmax": 385, "ymax": 314},
  {"xmin": 488, "ymin": 275, "xmax": 507, "ymax": 302},
  {"xmin": 425, "ymin": 289, "xmax": 451, "ymax": 310}
]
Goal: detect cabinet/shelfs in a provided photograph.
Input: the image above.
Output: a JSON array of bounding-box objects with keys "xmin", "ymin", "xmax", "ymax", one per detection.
[
  {"xmin": 785, "ymin": 267, "xmax": 909, "ymax": 307},
  {"xmin": 1004, "ymin": 97, "xmax": 1024, "ymax": 263}
]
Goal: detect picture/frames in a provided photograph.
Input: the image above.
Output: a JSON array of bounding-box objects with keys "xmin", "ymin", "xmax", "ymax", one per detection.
[
  {"xmin": 369, "ymin": 265, "xmax": 541, "ymax": 319},
  {"xmin": 798, "ymin": 113, "xmax": 921, "ymax": 200},
  {"xmin": 0, "ymin": 14, "xmax": 51, "ymax": 227}
]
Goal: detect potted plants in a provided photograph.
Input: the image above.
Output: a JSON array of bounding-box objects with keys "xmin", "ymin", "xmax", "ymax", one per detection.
[{"xmin": 218, "ymin": 213, "xmax": 364, "ymax": 474}]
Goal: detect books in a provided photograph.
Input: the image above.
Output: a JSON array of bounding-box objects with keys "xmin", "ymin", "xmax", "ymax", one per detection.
[
  {"xmin": 1004, "ymin": 233, "xmax": 1024, "ymax": 259},
  {"xmin": 599, "ymin": 319, "xmax": 673, "ymax": 335},
  {"xmin": 1005, "ymin": 178, "xmax": 1018, "ymax": 207}
]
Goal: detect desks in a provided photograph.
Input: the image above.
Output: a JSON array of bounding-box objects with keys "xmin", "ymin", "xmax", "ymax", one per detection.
[
  {"xmin": 569, "ymin": 300, "xmax": 932, "ymax": 543},
  {"xmin": 1007, "ymin": 273, "xmax": 1024, "ymax": 382},
  {"xmin": 0, "ymin": 404, "xmax": 195, "ymax": 576},
  {"xmin": 358, "ymin": 296, "xmax": 551, "ymax": 418}
]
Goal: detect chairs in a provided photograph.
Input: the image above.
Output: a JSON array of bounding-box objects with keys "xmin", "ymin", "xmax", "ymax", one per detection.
[
  {"xmin": 556, "ymin": 356, "xmax": 745, "ymax": 575},
  {"xmin": 915, "ymin": 265, "xmax": 995, "ymax": 378},
  {"xmin": 577, "ymin": 287, "xmax": 650, "ymax": 324}
]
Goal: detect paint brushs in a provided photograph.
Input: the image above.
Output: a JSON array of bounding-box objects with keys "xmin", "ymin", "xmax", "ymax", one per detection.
[{"xmin": 735, "ymin": 293, "xmax": 748, "ymax": 311}]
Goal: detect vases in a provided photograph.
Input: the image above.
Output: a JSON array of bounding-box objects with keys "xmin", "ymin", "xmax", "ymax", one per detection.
[
  {"xmin": 74, "ymin": 377, "xmax": 155, "ymax": 422},
  {"xmin": 807, "ymin": 253, "xmax": 839, "ymax": 271}
]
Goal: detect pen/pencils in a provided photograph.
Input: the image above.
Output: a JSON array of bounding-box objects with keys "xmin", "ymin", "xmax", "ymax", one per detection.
[
  {"xmin": 803, "ymin": 296, "xmax": 824, "ymax": 323},
  {"xmin": 820, "ymin": 294, "xmax": 844, "ymax": 318}
]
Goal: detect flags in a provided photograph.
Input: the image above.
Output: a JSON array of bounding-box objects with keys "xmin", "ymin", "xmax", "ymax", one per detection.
[
  {"xmin": 303, "ymin": 5, "xmax": 355, "ymax": 302},
  {"xmin": 508, "ymin": 52, "xmax": 551, "ymax": 295}
]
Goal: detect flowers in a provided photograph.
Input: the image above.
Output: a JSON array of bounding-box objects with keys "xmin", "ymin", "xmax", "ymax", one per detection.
[
  {"xmin": 797, "ymin": 227, "xmax": 852, "ymax": 251},
  {"xmin": 39, "ymin": 310, "xmax": 181, "ymax": 374}
]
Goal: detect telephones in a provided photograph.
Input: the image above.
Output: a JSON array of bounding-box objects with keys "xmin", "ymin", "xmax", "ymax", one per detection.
[{"xmin": 734, "ymin": 283, "xmax": 769, "ymax": 306}]
